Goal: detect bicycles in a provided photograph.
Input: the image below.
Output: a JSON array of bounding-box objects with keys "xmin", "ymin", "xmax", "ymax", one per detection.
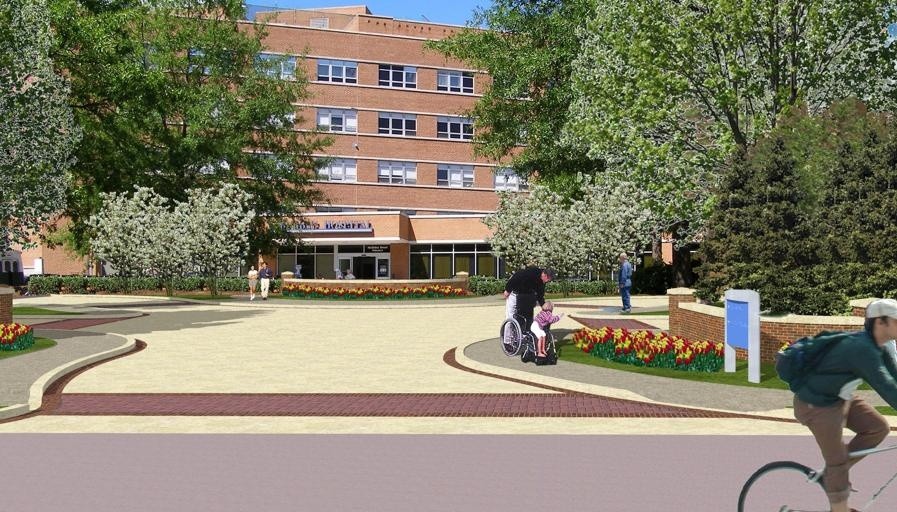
[{"xmin": 738, "ymin": 445, "xmax": 897, "ymax": 512}]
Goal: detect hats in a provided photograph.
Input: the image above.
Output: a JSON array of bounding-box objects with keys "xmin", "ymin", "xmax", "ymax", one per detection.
[{"xmin": 864, "ymin": 298, "xmax": 896, "ymax": 321}]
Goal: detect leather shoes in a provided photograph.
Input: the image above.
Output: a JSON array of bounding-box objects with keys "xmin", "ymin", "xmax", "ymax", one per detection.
[{"xmin": 502, "ymin": 344, "xmax": 512, "ymax": 351}]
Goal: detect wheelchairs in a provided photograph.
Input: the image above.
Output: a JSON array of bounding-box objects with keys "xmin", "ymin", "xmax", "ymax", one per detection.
[{"xmin": 500, "ymin": 292, "xmax": 558, "ymax": 365}]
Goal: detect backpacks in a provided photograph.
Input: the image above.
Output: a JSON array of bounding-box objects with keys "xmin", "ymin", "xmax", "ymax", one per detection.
[{"xmin": 775, "ymin": 331, "xmax": 862, "ymax": 392}]
